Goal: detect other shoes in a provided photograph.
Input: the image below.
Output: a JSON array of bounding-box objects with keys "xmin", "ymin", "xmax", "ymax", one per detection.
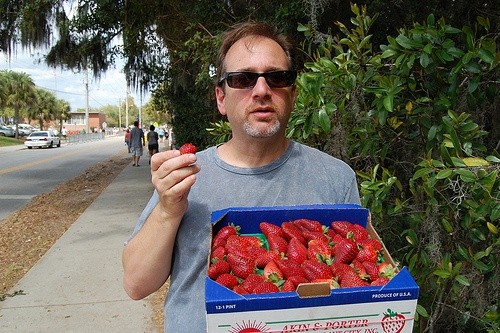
[
  {"xmin": 132, "ymin": 164, "xmax": 135, "ymax": 166},
  {"xmin": 137, "ymin": 165, "xmax": 140, "ymax": 167},
  {"xmin": 149, "ymin": 161, "xmax": 151, "ymax": 165}
]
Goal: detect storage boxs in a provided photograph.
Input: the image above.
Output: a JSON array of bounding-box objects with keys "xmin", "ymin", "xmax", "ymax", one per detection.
[{"xmin": 204, "ymin": 204, "xmax": 419, "ymax": 333}]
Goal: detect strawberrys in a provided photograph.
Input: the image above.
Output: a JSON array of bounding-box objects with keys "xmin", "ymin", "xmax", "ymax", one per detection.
[
  {"xmin": 208, "ymin": 219, "xmax": 397, "ymax": 294},
  {"xmin": 179, "ymin": 143, "xmax": 197, "ymax": 155}
]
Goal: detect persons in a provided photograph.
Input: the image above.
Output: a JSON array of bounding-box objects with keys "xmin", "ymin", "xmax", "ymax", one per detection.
[
  {"xmin": 62, "ymin": 126, "xmax": 68, "ymax": 143},
  {"xmin": 159, "ymin": 127, "xmax": 164, "ymax": 142},
  {"xmin": 125, "ymin": 129, "xmax": 131, "ymax": 153},
  {"xmin": 147, "ymin": 125, "xmax": 159, "ymax": 160},
  {"xmin": 123, "ymin": 20, "xmax": 361, "ymax": 333},
  {"xmin": 128, "ymin": 120, "xmax": 145, "ymax": 167}
]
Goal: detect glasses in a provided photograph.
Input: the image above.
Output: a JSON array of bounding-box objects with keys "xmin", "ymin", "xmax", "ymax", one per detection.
[{"xmin": 217, "ymin": 69, "xmax": 298, "ymax": 90}]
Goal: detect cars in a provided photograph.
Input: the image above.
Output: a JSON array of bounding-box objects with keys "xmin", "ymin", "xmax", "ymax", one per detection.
[
  {"xmin": 155, "ymin": 127, "xmax": 165, "ymax": 136},
  {"xmin": 24, "ymin": 131, "xmax": 62, "ymax": 149},
  {"xmin": 0, "ymin": 124, "xmax": 59, "ymax": 137}
]
